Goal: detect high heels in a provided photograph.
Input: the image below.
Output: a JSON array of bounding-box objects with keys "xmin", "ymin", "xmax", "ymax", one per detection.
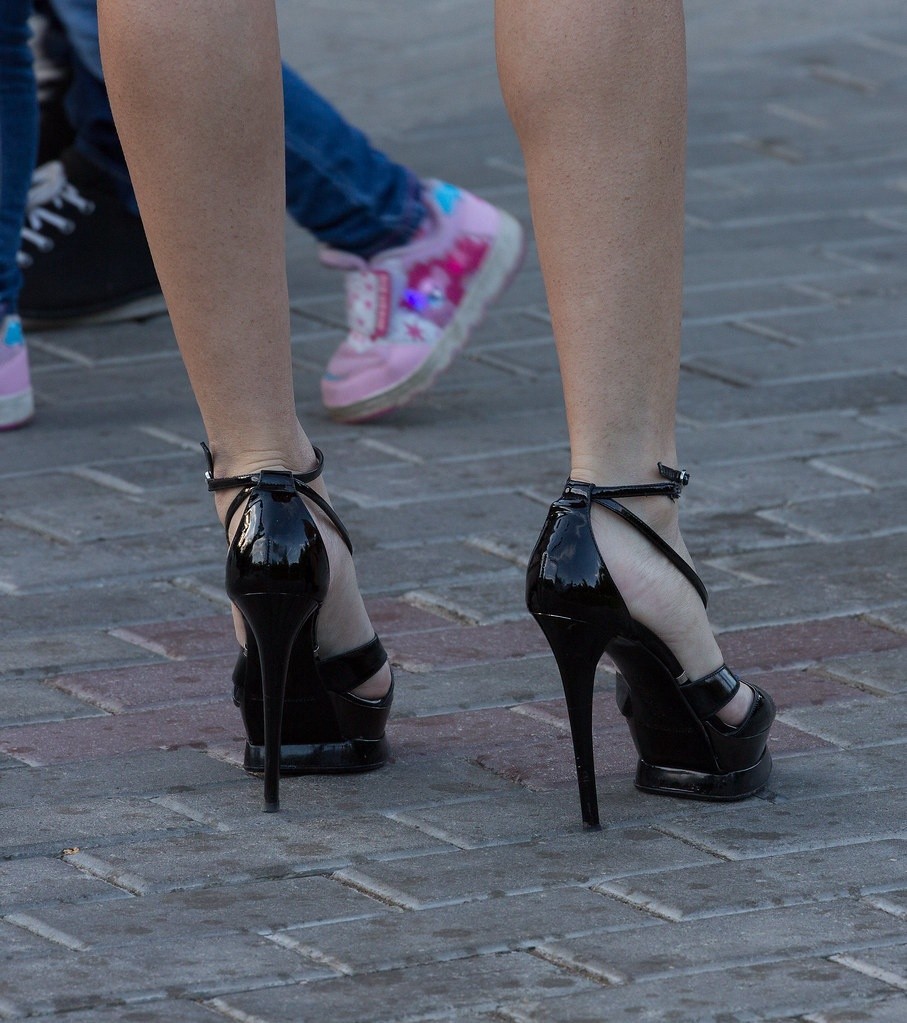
[
  {"xmin": 525, "ymin": 459, "xmax": 775, "ymax": 831},
  {"xmin": 200, "ymin": 441, "xmax": 395, "ymax": 812}
]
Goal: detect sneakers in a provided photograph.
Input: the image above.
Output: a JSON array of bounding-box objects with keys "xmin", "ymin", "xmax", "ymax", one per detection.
[
  {"xmin": 317, "ymin": 174, "xmax": 527, "ymax": 426},
  {"xmin": 0, "ymin": 314, "xmax": 38, "ymax": 430},
  {"xmin": 27, "ymin": 0, "xmax": 79, "ymax": 168},
  {"xmin": 16, "ymin": 149, "xmax": 169, "ymax": 332}
]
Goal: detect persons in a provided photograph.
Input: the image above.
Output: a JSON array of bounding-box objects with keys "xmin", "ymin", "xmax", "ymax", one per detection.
[
  {"xmin": 96, "ymin": 0, "xmax": 777, "ymax": 829},
  {"xmin": 0, "ymin": 0, "xmax": 526, "ymax": 432}
]
